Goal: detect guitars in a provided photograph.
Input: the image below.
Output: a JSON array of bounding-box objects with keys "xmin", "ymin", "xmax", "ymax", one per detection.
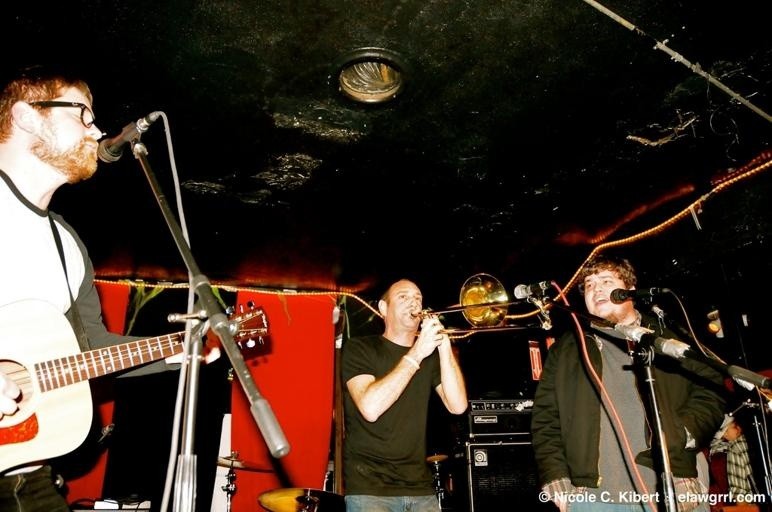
[{"xmin": 0, "ymin": 298, "xmax": 269, "ymax": 474}]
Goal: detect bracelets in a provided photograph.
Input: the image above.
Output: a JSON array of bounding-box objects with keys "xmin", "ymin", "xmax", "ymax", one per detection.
[{"xmin": 403, "ymin": 355, "xmax": 420, "ymax": 370}]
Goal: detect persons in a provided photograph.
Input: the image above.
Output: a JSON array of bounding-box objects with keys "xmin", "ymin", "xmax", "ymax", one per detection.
[
  {"xmin": 341, "ymin": 279, "xmax": 468, "ymax": 512},
  {"xmin": 0, "ymin": 66, "xmax": 182, "ymax": 512},
  {"xmin": 531, "ymin": 256, "xmax": 728, "ymax": 512}
]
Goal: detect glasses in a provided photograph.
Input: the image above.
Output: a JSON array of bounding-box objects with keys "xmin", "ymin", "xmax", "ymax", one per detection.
[
  {"xmin": 30, "ymin": 100, "xmax": 96, "ymax": 128},
  {"xmin": 578, "ymin": 280, "xmax": 596, "ymax": 293}
]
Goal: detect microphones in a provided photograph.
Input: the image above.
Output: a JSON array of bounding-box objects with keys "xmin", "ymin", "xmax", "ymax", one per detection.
[
  {"xmin": 513, "ymin": 280, "xmax": 547, "ymax": 301},
  {"xmin": 609, "ymin": 287, "xmax": 657, "ymax": 305},
  {"xmin": 97, "ymin": 111, "xmax": 158, "ymax": 165}
]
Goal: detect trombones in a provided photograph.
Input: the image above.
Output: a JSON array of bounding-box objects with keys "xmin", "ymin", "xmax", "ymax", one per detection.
[{"xmin": 409, "ymin": 274, "xmax": 551, "ymax": 336}]
